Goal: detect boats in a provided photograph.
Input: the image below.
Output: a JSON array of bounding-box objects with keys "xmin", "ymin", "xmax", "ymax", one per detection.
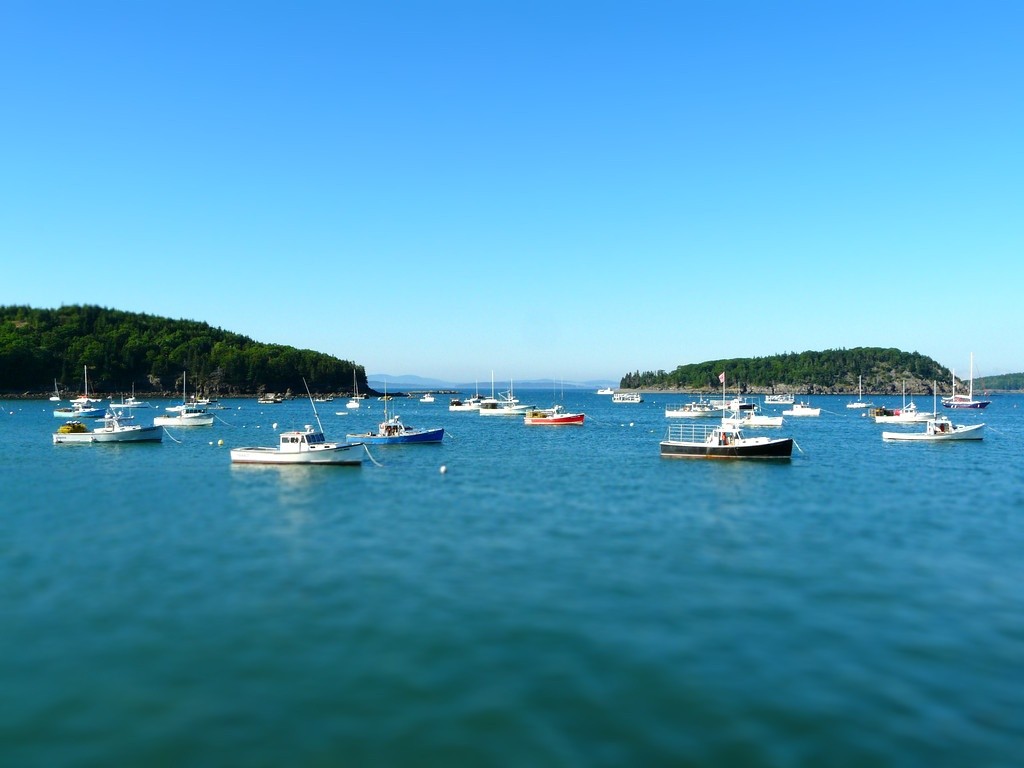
[
  {"xmin": 782, "ymin": 399, "xmax": 821, "ymax": 418},
  {"xmin": 664, "ymin": 393, "xmax": 723, "ymax": 418},
  {"xmin": 597, "ymin": 387, "xmax": 615, "ymax": 395},
  {"xmin": 723, "ymin": 400, "xmax": 784, "ymax": 425},
  {"xmin": 874, "ymin": 379, "xmax": 942, "ymax": 423},
  {"xmin": 257, "ymin": 396, "xmax": 282, "ymax": 405},
  {"xmin": 447, "ymin": 379, "xmax": 481, "ymax": 411},
  {"xmin": 882, "ymin": 379, "xmax": 986, "ymax": 442},
  {"xmin": 611, "ymin": 392, "xmax": 644, "ymax": 403},
  {"xmin": 378, "ymin": 395, "xmax": 394, "ymax": 402},
  {"xmin": 709, "ymin": 397, "xmax": 756, "ymax": 410},
  {"xmin": 524, "ymin": 408, "xmax": 585, "ymax": 426},
  {"xmin": 420, "ymin": 393, "xmax": 435, "ymax": 403},
  {"xmin": 49, "ymin": 362, "xmax": 217, "ymax": 444},
  {"xmin": 351, "ymin": 396, "xmax": 365, "ymax": 399},
  {"xmin": 346, "ymin": 379, "xmax": 445, "ymax": 446},
  {"xmin": 230, "ymin": 377, "xmax": 366, "ymax": 466},
  {"xmin": 314, "ymin": 397, "xmax": 333, "ymax": 402},
  {"xmin": 764, "ymin": 393, "xmax": 796, "ymax": 405},
  {"xmin": 335, "ymin": 412, "xmax": 348, "ymax": 416}
]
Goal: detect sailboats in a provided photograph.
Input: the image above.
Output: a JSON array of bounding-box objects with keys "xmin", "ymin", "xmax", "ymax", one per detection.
[
  {"xmin": 847, "ymin": 375, "xmax": 873, "ymax": 408},
  {"xmin": 659, "ymin": 372, "xmax": 794, "ymax": 460},
  {"xmin": 478, "ymin": 370, "xmax": 537, "ymax": 417},
  {"xmin": 345, "ymin": 368, "xmax": 360, "ymax": 408},
  {"xmin": 940, "ymin": 352, "xmax": 992, "ymax": 408}
]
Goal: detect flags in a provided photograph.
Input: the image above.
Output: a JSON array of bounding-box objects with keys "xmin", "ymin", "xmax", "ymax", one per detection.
[{"xmin": 719, "ymin": 372, "xmax": 725, "ymax": 383}]
